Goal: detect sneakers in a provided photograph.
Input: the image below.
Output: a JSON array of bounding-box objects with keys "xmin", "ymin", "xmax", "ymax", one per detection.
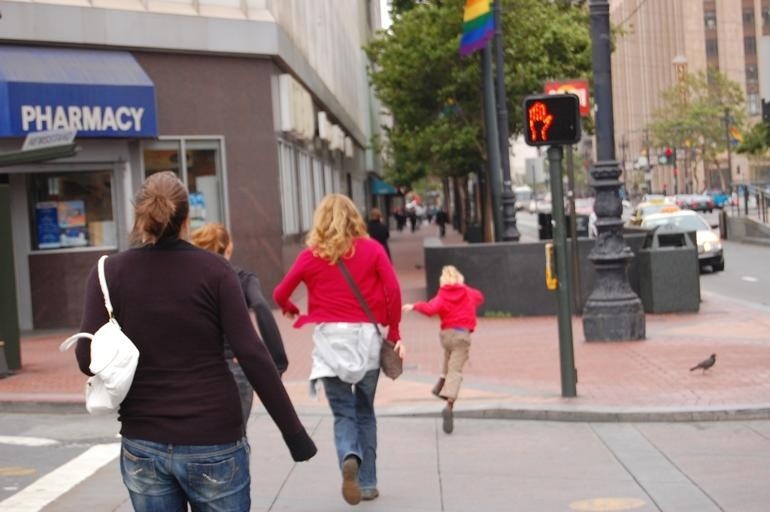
[
  {"xmin": 442, "ymin": 407, "xmax": 453, "ymax": 433},
  {"xmin": 342, "ymin": 458, "xmax": 378, "ymax": 504},
  {"xmin": 432, "ymin": 379, "xmax": 448, "ymax": 400}
]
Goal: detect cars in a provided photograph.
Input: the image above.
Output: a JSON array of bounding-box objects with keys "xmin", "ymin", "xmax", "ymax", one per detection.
[{"xmin": 512, "ymin": 184, "xmax": 739, "ymax": 275}]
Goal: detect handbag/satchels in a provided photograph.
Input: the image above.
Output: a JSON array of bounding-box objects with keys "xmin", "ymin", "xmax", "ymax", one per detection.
[
  {"xmin": 381, "ymin": 340, "xmax": 402, "ymax": 379},
  {"xmin": 59, "ymin": 319, "xmax": 139, "ymax": 415}
]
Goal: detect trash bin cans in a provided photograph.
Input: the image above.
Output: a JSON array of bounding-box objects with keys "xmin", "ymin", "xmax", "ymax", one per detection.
[{"xmin": 637, "ymin": 224, "xmax": 700, "ymax": 314}]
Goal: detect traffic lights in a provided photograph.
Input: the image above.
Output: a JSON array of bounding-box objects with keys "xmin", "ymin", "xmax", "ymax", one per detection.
[
  {"xmin": 521, "ymin": 94, "xmax": 582, "ymax": 146},
  {"xmin": 664, "ymin": 146, "xmax": 673, "ymax": 165}
]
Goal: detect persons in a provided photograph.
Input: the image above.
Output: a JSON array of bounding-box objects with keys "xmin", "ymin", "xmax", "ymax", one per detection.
[
  {"xmin": 426, "ymin": 209, "xmax": 433, "ymax": 224},
  {"xmin": 436, "ymin": 206, "xmax": 450, "ymax": 238},
  {"xmin": 270, "ymin": 192, "xmax": 405, "ymax": 508},
  {"xmin": 74, "ymin": 170, "xmax": 319, "ymax": 512},
  {"xmin": 408, "ymin": 207, "xmax": 420, "ymax": 233},
  {"xmin": 402, "ymin": 263, "xmax": 486, "ymax": 437},
  {"xmin": 365, "ymin": 206, "xmax": 390, "ymax": 261},
  {"xmin": 395, "ymin": 205, "xmax": 408, "ymax": 232},
  {"xmin": 191, "ymin": 218, "xmax": 289, "ymax": 437}
]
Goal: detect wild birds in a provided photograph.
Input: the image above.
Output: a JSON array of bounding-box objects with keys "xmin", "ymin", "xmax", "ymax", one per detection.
[{"xmin": 689, "ymin": 354, "xmax": 716, "ymax": 372}]
